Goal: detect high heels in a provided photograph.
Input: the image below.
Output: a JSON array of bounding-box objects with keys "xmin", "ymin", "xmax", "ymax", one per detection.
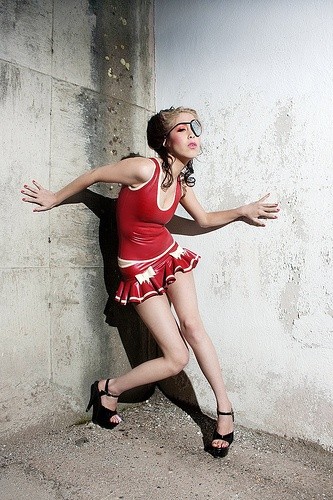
[
  {"xmin": 210, "ymin": 407, "xmax": 235, "ymax": 457},
  {"xmin": 86, "ymin": 379, "xmax": 119, "ymax": 429}
]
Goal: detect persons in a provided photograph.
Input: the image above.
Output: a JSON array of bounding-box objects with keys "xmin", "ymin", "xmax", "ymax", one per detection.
[{"xmin": 21, "ymin": 106, "xmax": 279, "ymax": 458}]
